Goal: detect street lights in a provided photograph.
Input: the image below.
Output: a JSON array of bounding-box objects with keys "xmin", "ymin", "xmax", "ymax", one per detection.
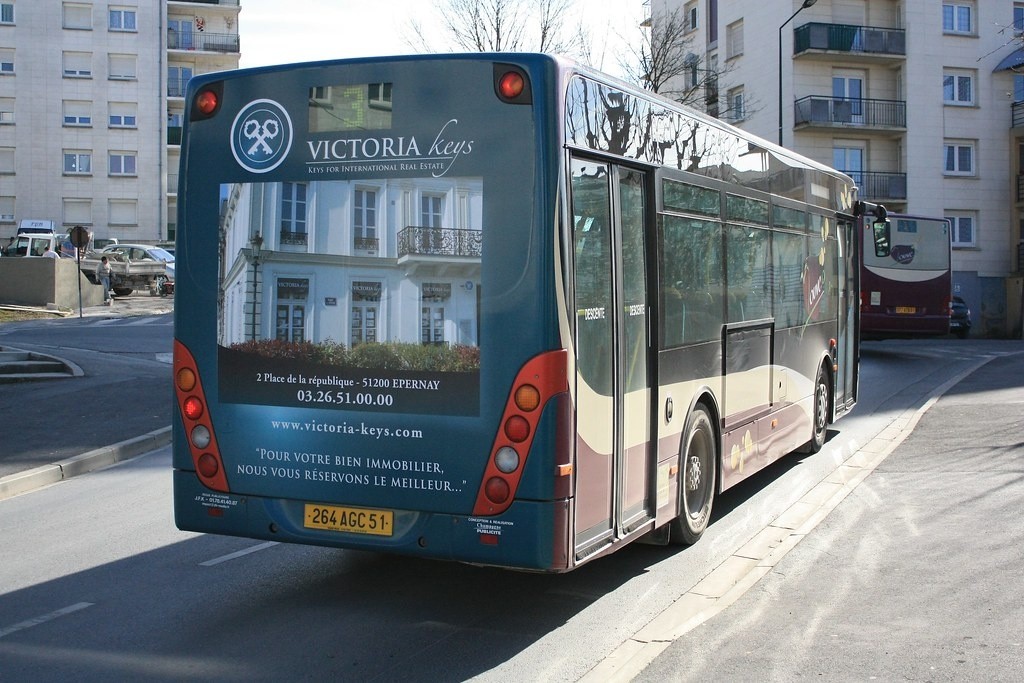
[{"xmin": 777, "ymin": 0, "xmax": 819, "ymax": 149}]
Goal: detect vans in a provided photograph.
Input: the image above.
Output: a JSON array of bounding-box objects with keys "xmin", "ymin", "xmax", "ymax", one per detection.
[
  {"xmin": 12, "ymin": 219, "xmax": 56, "ymax": 252},
  {"xmin": 101, "ymin": 241, "xmax": 176, "ymax": 294}
]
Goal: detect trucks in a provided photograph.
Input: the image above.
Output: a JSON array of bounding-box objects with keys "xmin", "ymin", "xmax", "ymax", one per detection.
[{"xmin": 0, "ymin": 228, "xmax": 166, "ymax": 297}]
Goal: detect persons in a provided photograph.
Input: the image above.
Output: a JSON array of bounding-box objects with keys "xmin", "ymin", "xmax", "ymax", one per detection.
[
  {"xmin": 96, "ymin": 256, "xmax": 114, "ymax": 301},
  {"xmin": 42, "ymin": 246, "xmax": 60, "ymax": 258}
]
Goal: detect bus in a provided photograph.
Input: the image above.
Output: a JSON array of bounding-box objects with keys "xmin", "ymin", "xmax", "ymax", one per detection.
[
  {"xmin": 174, "ymin": 51, "xmax": 892, "ymax": 578},
  {"xmin": 801, "ymin": 210, "xmax": 954, "ymax": 343}
]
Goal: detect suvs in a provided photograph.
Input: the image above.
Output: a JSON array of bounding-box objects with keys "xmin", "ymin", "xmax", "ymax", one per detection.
[{"xmin": 93, "ymin": 237, "xmax": 120, "ymax": 255}]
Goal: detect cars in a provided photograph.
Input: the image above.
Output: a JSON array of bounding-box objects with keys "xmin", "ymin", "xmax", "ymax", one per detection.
[{"xmin": 949, "ymin": 295, "xmax": 972, "ymax": 340}]
